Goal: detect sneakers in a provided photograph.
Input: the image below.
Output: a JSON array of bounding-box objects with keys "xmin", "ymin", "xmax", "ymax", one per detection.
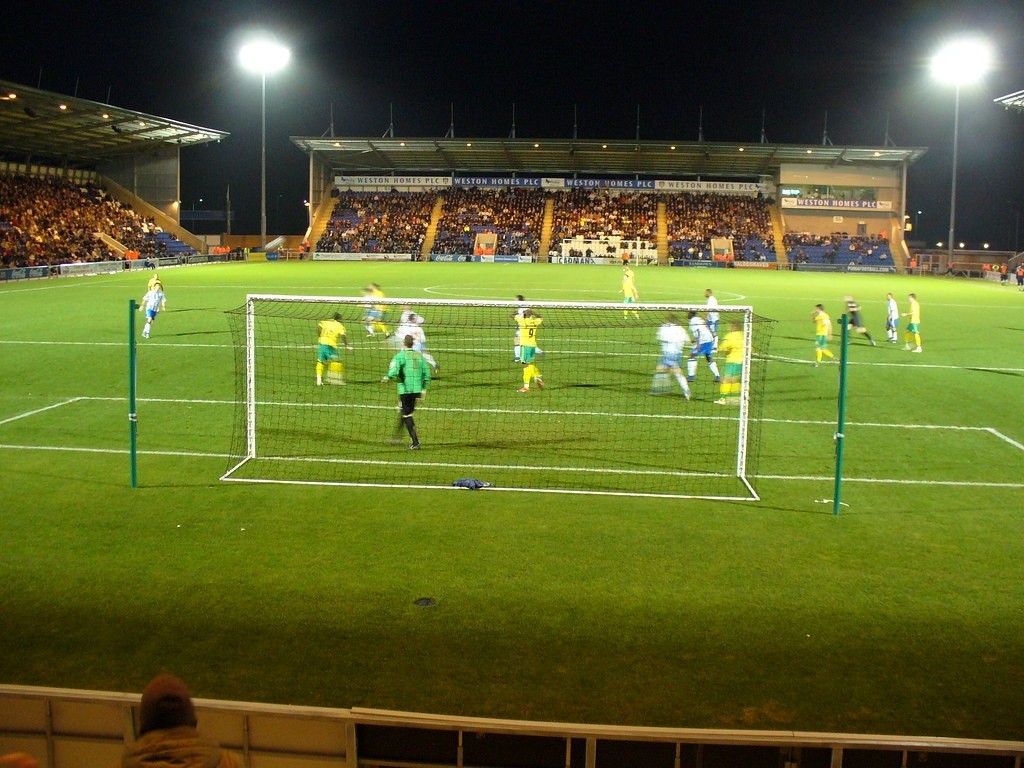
[
  {"xmin": 142, "ymin": 333, "xmax": 146, "ymax": 337},
  {"xmin": 409, "ymin": 444, "xmax": 420, "ymax": 450},
  {"xmin": 146, "ymin": 334, "xmax": 150, "ymax": 338}
]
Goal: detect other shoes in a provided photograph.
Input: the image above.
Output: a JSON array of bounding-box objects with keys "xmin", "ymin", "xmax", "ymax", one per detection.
[
  {"xmin": 518, "ymin": 387, "xmax": 529, "ymax": 391},
  {"xmin": 687, "ymin": 376, "xmax": 694, "ymax": 381},
  {"xmin": 713, "ymin": 376, "xmax": 720, "ymax": 382},
  {"xmin": 912, "ymin": 347, "xmax": 922, "ymax": 352},
  {"xmin": 902, "ymin": 346, "xmax": 912, "ymax": 351}
]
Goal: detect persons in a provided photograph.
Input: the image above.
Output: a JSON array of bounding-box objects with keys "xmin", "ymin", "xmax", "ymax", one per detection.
[
  {"xmin": 686, "ymin": 310, "xmax": 720, "ymax": 382},
  {"xmin": 714, "ymin": 320, "xmax": 754, "ymax": 405},
  {"xmin": 356, "ymin": 283, "xmax": 439, "ymax": 374},
  {"xmin": 704, "ymin": 289, "xmax": 720, "ymax": 352},
  {"xmin": 618, "ymin": 274, "xmax": 640, "ymax": 319},
  {"xmin": 147, "ymin": 274, "xmax": 163, "ymax": 314},
  {"xmin": 648, "ymin": 314, "xmax": 695, "ymax": 401},
  {"xmin": 844, "ymin": 292, "xmax": 877, "ymax": 347},
  {"xmin": 512, "ymin": 295, "xmax": 544, "ymax": 362},
  {"xmin": 122, "ymin": 672, "xmax": 238, "ymax": 768},
  {"xmin": 900, "ymin": 294, "xmax": 922, "ymax": 353},
  {"xmin": 811, "ymin": 304, "xmax": 839, "ymax": 368},
  {"xmin": 139, "ymin": 283, "xmax": 167, "ymax": 339},
  {"xmin": 277, "ymin": 186, "xmax": 1024, "ymax": 288},
  {"xmin": 509, "ymin": 310, "xmax": 544, "ymax": 393},
  {"xmin": 0, "ymin": 168, "xmax": 246, "ymax": 279},
  {"xmin": 379, "ymin": 334, "xmax": 430, "ymax": 450},
  {"xmin": 315, "ymin": 313, "xmax": 353, "ymax": 387},
  {"xmin": 885, "ymin": 292, "xmax": 899, "ymax": 344}
]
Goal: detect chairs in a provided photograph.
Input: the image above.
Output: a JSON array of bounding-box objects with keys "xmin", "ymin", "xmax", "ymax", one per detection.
[
  {"xmin": 317, "ymin": 191, "xmax": 896, "ymax": 266},
  {"xmin": 143, "ymin": 262, "xmax": 151, "ymax": 270},
  {"xmin": 0, "ymin": 222, "xmax": 12, "ymax": 230},
  {"xmin": 118, "ymin": 222, "xmax": 199, "ymax": 257}
]
